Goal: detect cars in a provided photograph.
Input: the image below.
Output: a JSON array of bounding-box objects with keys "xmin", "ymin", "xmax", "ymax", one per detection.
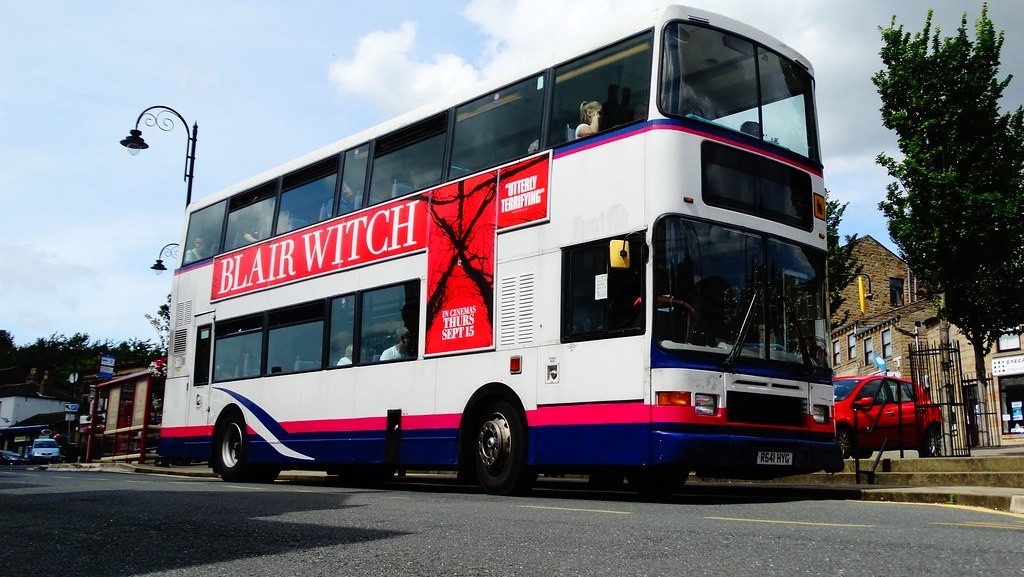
[
  {"xmin": 825, "ymin": 376, "xmax": 947, "ymax": 460},
  {"xmin": 0, "ymin": 450, "xmax": 23, "ymax": 464},
  {"xmin": 31, "ymin": 438, "xmax": 60, "ymax": 463}
]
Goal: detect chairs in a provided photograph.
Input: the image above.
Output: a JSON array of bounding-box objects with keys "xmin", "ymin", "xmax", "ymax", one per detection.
[
  {"xmin": 234, "ymin": 354, "xmax": 385, "ymax": 378},
  {"xmin": 209, "ymin": 164, "xmax": 476, "ymax": 257}
]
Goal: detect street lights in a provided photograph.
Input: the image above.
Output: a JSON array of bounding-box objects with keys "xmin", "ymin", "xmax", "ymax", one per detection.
[{"xmin": 119, "ymin": 105, "xmax": 199, "ymax": 210}]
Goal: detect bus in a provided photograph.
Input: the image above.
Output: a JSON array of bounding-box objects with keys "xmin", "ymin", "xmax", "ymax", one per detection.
[{"xmin": 156, "ymin": 4, "xmax": 845, "ymax": 507}]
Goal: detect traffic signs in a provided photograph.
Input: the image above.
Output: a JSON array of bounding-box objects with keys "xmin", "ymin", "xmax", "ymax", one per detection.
[
  {"xmin": 98, "ymin": 354, "xmax": 115, "ymax": 379},
  {"xmin": 64, "ymin": 404, "xmax": 78, "ymax": 412}
]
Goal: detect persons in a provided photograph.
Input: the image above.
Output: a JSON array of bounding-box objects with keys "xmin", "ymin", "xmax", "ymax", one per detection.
[
  {"xmin": 324, "ymin": 172, "xmax": 360, "ymax": 218},
  {"xmin": 336, "ymin": 328, "xmax": 416, "ymax": 365},
  {"xmin": 740, "ymin": 121, "xmax": 765, "ymax": 142},
  {"xmin": 183, "ymin": 235, "xmax": 210, "ymax": 266},
  {"xmin": 576, "ymin": 99, "xmax": 604, "ymax": 139},
  {"xmin": 236, "ymin": 219, "xmax": 259, "ymax": 248}
]
